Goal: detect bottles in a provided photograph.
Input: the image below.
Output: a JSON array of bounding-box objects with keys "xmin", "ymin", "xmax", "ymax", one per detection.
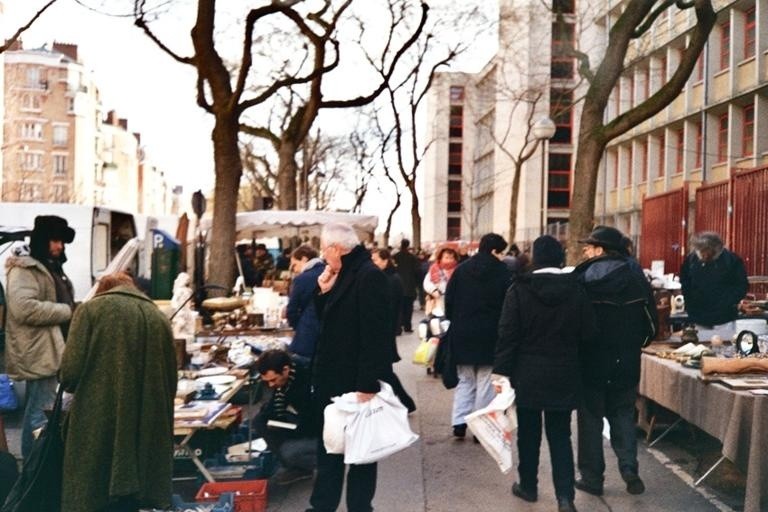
[{"xmin": 679, "ymin": 329, "xmax": 699, "ymax": 345}]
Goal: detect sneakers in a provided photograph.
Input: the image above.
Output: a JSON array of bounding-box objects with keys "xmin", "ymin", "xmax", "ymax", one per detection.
[
  {"xmin": 272, "ymin": 467, "xmax": 314, "ymax": 486},
  {"xmin": 558, "ymin": 495, "xmax": 578, "ymax": 512},
  {"xmin": 624, "ymin": 471, "xmax": 646, "ymax": 495},
  {"xmin": 574, "ymin": 478, "xmax": 604, "ymax": 497},
  {"xmin": 453, "ymin": 425, "xmax": 466, "ymax": 437},
  {"xmin": 512, "ymin": 481, "xmax": 537, "ymax": 503}
]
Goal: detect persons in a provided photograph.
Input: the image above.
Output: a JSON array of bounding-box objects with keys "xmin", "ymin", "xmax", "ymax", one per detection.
[
  {"xmin": 280, "ymin": 243, "xmax": 328, "ymax": 359},
  {"xmin": 623, "ymin": 236, "xmax": 633, "ymax": 255},
  {"xmin": 237, "ymin": 235, "xmax": 302, "ymax": 288},
  {"xmin": 493, "ymin": 234, "xmax": 604, "ymax": 511},
  {"xmin": 245, "ymin": 347, "xmax": 322, "ymax": 487},
  {"xmin": 3, "ymin": 215, "xmax": 85, "ymax": 472},
  {"xmin": 305, "ymin": 221, "xmax": 401, "ymax": 511},
  {"xmin": 368, "ymin": 238, "xmax": 469, "ymax": 341},
  {"xmin": 676, "ymin": 231, "xmax": 750, "ymax": 345},
  {"xmin": 55, "ymin": 271, "xmax": 178, "ymax": 511},
  {"xmin": 2, "ymin": 280, "xmax": 10, "ymax": 455},
  {"xmin": 567, "ymin": 225, "xmax": 661, "ymax": 496},
  {"xmin": 228, "ymin": 453, "xmax": 253, "ymax": 462},
  {"xmin": 443, "ymin": 231, "xmax": 517, "ymax": 444}
]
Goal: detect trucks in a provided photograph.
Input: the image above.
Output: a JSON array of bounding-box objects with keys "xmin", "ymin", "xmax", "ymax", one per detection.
[{"xmin": 1, "ymin": 198, "xmax": 190, "ymax": 308}]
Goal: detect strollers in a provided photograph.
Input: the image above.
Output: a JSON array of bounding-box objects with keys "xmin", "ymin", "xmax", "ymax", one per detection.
[{"xmin": 423, "ymin": 296, "xmax": 452, "ymax": 375}]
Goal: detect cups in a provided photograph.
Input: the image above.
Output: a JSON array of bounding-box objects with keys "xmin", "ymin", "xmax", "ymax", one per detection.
[{"xmin": 263, "ymin": 305, "xmax": 281, "ymax": 329}]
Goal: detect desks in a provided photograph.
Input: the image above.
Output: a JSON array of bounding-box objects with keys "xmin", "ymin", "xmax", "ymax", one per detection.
[
  {"xmin": 172, "ymin": 373, "xmax": 250, "ymax": 483},
  {"xmin": 639, "ymin": 346, "xmax": 768, "ymax": 510}
]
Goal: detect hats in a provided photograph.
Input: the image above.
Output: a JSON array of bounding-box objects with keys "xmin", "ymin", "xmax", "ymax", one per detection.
[
  {"xmin": 576, "ymin": 224, "xmax": 632, "ymax": 251},
  {"xmin": 94, "ymin": 271, "xmax": 136, "ymax": 297},
  {"xmin": 692, "ymin": 231, "xmax": 723, "ymax": 251},
  {"xmin": 531, "ymin": 234, "xmax": 565, "ymax": 268},
  {"xmin": 28, "ymin": 215, "xmax": 76, "ymax": 245}
]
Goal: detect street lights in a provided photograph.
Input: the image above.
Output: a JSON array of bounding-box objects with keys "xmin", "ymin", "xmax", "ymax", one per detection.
[{"xmin": 532, "ymin": 112, "xmax": 556, "ymax": 237}]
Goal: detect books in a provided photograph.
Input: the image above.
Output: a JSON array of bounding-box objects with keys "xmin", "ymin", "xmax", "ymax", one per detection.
[
  {"xmin": 171, "ymin": 406, "xmax": 207, "ymax": 419},
  {"xmin": 172, "ymin": 398, "xmax": 233, "ymax": 428}
]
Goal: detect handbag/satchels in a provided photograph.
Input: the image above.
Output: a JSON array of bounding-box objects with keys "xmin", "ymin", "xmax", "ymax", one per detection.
[
  {"xmin": 322, "ymin": 377, "xmax": 421, "ymax": 466},
  {"xmin": 0, "ymin": 382, "xmax": 72, "ymax": 511},
  {"xmin": 437, "ymin": 318, "xmax": 461, "ymax": 391},
  {"xmin": 412, "ymin": 335, "xmax": 441, "ymax": 369},
  {"xmin": 0, "ymin": 374, "xmax": 19, "ymax": 412},
  {"xmin": 463, "ymin": 375, "xmax": 519, "ymax": 476}
]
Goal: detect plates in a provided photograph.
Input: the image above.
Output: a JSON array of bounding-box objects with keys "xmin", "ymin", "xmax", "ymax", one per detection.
[{"xmin": 173, "ymin": 365, "xmax": 239, "ymax": 400}]
